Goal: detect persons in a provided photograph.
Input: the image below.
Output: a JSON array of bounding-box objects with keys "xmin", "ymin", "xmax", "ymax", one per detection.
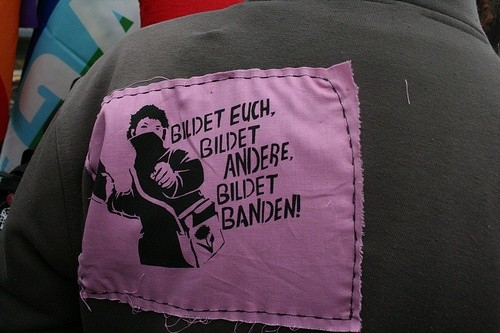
[{"xmin": 0, "ymin": 0, "xmax": 500, "ymax": 333}]
